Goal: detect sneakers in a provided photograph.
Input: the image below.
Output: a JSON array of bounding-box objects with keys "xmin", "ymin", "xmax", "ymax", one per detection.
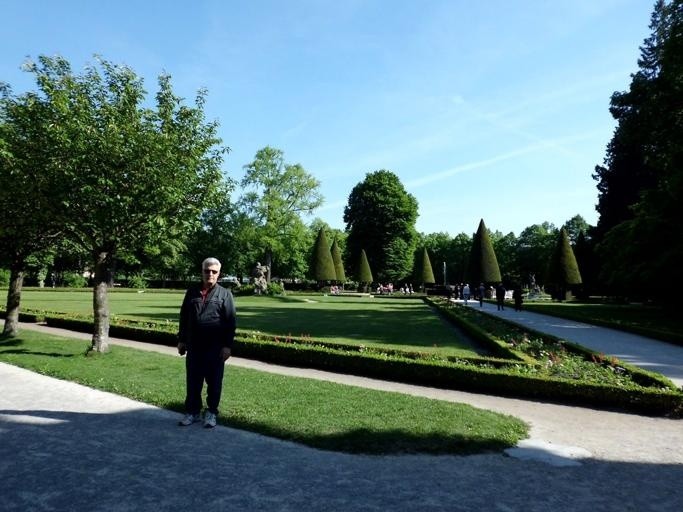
[
  {"xmin": 179, "ymin": 413, "xmax": 201, "ymax": 426},
  {"xmin": 204, "ymin": 411, "xmax": 217, "ymax": 427}
]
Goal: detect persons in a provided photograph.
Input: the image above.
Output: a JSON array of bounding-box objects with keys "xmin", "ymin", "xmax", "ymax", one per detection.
[
  {"xmin": 446, "ymin": 282, "xmax": 523, "ymax": 312},
  {"xmin": 331, "ymin": 285, "xmax": 339, "ymax": 293},
  {"xmin": 177, "ymin": 257, "xmax": 240, "ymax": 427},
  {"xmin": 376, "ymin": 282, "xmax": 414, "ymax": 295}
]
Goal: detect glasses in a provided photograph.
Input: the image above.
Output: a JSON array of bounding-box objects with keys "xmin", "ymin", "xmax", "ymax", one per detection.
[{"xmin": 204, "ymin": 269, "xmax": 218, "ymax": 274}]
